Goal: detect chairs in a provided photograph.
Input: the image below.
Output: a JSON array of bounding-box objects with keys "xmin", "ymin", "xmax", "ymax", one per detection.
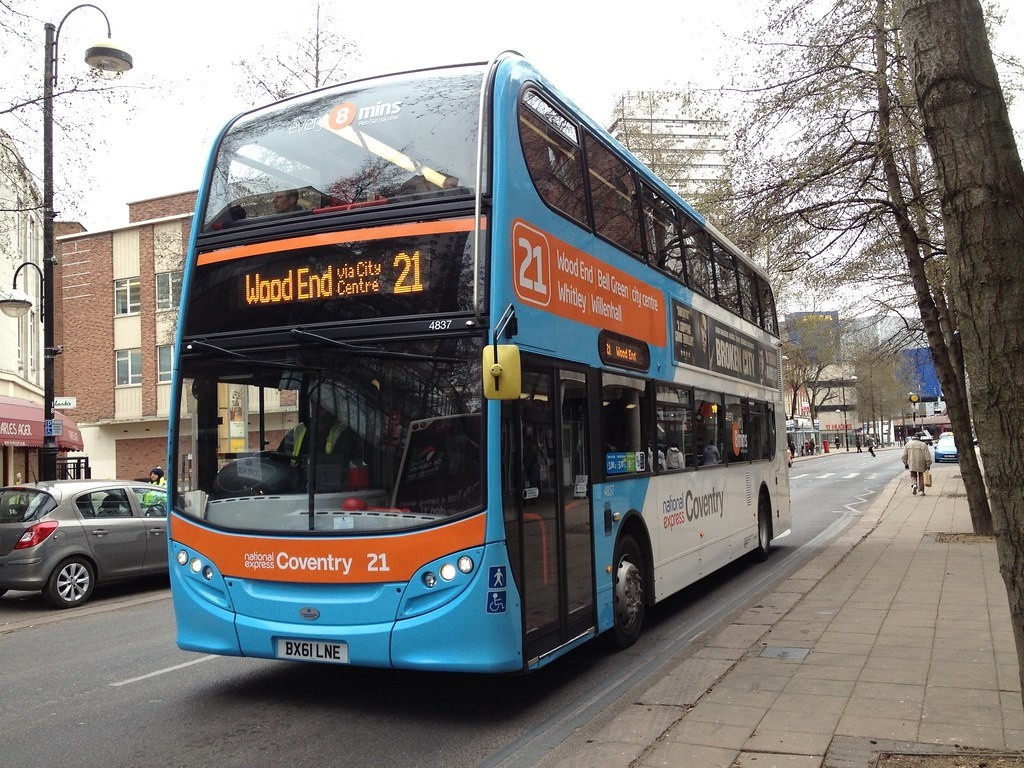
[{"xmin": 98, "ymin": 494, "xmax": 123, "ymax": 515}]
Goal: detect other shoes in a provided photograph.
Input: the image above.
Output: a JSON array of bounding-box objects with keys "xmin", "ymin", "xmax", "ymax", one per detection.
[
  {"xmin": 919, "ymin": 491, "xmax": 925, "ymax": 496},
  {"xmin": 912, "ymin": 485, "xmax": 917, "ymax": 495}
]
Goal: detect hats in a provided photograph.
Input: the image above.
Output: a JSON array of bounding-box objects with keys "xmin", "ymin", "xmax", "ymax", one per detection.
[
  {"xmin": 150, "ymin": 466, "xmax": 164, "ymax": 484},
  {"xmin": 914, "ymin": 432, "xmax": 921, "ymax": 437}
]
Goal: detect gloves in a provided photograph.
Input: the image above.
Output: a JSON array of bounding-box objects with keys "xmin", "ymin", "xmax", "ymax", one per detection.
[
  {"xmin": 905, "ymin": 464, "xmax": 909, "ymax": 469},
  {"xmin": 926, "ymin": 467, "xmax": 930, "ymax": 470}
]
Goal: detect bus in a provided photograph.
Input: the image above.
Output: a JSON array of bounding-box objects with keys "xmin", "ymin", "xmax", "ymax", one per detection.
[{"xmin": 163, "ymin": 46, "xmax": 792, "ymax": 680}]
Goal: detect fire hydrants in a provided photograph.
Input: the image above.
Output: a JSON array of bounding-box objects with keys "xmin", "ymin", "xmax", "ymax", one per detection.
[{"xmin": 823, "ymin": 440, "xmax": 830, "ymax": 454}]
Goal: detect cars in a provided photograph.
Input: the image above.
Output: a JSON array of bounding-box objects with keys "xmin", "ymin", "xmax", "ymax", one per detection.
[
  {"xmin": 0, "ymin": 478, "xmax": 169, "ymax": 611},
  {"xmin": 933, "ymin": 435, "xmax": 960, "ymax": 463}
]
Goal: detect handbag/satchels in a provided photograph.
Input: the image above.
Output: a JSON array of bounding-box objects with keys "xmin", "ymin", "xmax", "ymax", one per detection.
[{"xmin": 923, "ymin": 469, "xmax": 932, "ymax": 487}]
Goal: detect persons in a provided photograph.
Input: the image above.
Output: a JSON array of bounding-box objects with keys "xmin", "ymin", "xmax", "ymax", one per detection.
[
  {"xmin": 144, "ymin": 466, "xmax": 168, "ymax": 507},
  {"xmin": 274, "ymin": 382, "xmax": 369, "ymax": 469},
  {"xmin": 835, "ymin": 436, "xmax": 840, "ymax": 449},
  {"xmin": 270, "ymin": 182, "xmax": 305, "ymax": 214},
  {"xmin": 525, "ymin": 433, "xmax": 543, "ymax": 505},
  {"xmin": 647, "ymin": 438, "xmax": 724, "ymax": 473},
  {"xmin": 787, "ymin": 441, "xmax": 795, "ymax": 468},
  {"xmin": 901, "ymin": 432, "xmax": 932, "ymax": 496},
  {"xmin": 806, "ymin": 438, "xmax": 815, "ymax": 455},
  {"xmin": 856, "ymin": 437, "xmax": 863, "ymax": 453},
  {"xmin": 866, "ymin": 434, "xmax": 877, "ymax": 458}
]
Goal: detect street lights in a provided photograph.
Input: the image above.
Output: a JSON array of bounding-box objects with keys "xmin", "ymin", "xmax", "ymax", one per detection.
[
  {"xmin": 835, "ymin": 375, "xmax": 858, "ymax": 452},
  {"xmin": 0, "ymin": 3, "xmax": 135, "ymax": 481}
]
